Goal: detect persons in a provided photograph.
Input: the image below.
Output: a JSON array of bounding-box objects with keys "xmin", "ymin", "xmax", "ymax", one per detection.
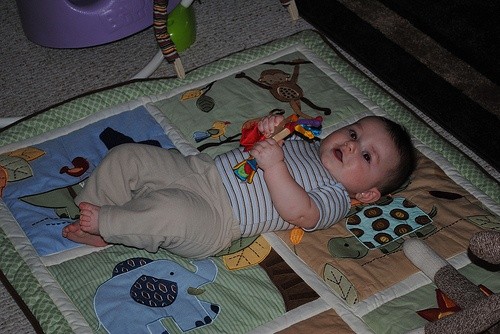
[{"xmin": 63, "ymin": 116, "xmax": 417, "ymax": 261}]
